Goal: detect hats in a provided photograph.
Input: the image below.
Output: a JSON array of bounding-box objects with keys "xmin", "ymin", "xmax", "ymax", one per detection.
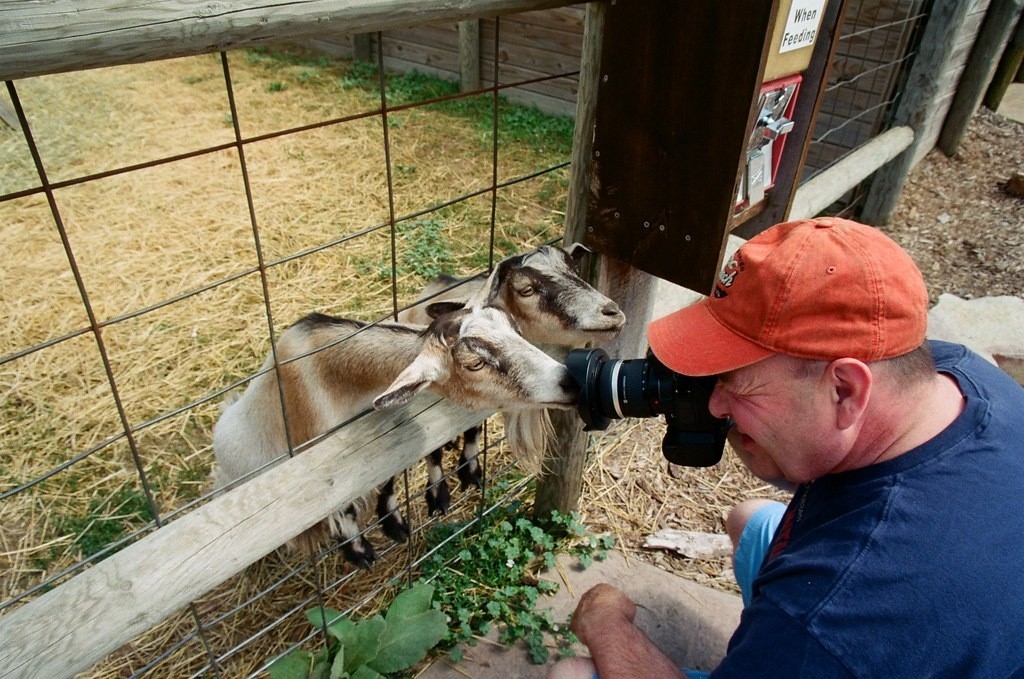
[{"xmin": 647, "ymin": 216, "xmax": 927, "ymax": 376}]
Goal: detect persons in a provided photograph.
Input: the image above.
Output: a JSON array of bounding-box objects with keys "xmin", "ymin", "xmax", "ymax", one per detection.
[{"xmin": 542, "ymin": 217, "xmax": 1024, "ymax": 679}]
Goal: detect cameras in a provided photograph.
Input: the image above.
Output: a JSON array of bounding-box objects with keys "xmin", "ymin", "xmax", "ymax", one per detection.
[{"xmin": 566, "ymin": 345, "xmax": 731, "ymax": 468}]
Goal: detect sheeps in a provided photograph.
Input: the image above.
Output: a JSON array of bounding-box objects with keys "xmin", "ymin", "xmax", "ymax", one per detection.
[{"xmin": 213, "ymin": 242, "xmax": 626, "ymax": 572}]
